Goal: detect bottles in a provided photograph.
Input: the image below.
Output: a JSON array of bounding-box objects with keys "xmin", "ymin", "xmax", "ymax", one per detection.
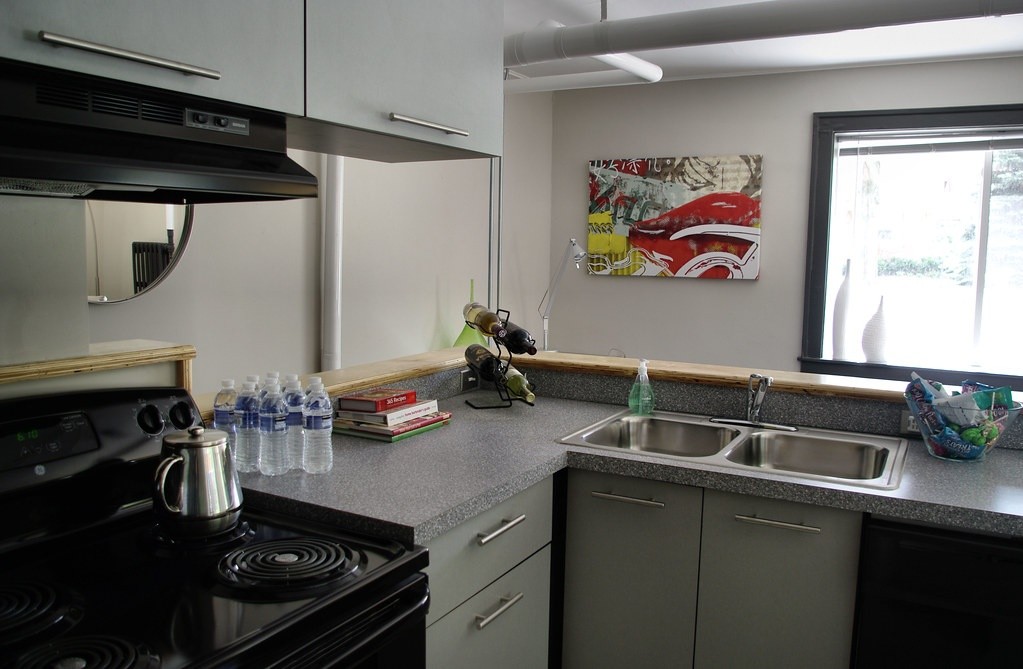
[
  {"xmin": 495, "ymin": 318, "xmax": 537, "ymax": 355},
  {"xmin": 499, "ymin": 359, "xmax": 536, "ymax": 402},
  {"xmin": 463, "ymin": 302, "xmax": 507, "ymax": 338},
  {"xmin": 465, "ymin": 343, "xmax": 508, "ymax": 385},
  {"xmin": 214, "ymin": 371, "xmax": 334, "ymax": 477}
]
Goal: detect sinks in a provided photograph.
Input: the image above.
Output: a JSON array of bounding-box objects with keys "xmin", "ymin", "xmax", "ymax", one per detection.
[
  {"xmin": 708, "ymin": 423, "xmax": 908, "ymax": 489},
  {"xmin": 553, "ymin": 408, "xmax": 749, "ymax": 466}
]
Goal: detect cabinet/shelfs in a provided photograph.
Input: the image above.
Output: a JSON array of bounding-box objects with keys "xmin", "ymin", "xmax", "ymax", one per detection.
[
  {"xmin": 562, "ymin": 467, "xmax": 860, "ymax": 669},
  {"xmin": 306, "ymin": 1, "xmax": 504, "ymax": 164},
  {"xmin": 421, "ymin": 476, "xmax": 554, "ymax": 669},
  {"xmin": 0, "ymin": 0, "xmax": 305, "ymax": 119}
]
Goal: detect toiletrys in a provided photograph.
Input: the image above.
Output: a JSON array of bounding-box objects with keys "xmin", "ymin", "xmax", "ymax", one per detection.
[{"xmin": 628, "ymin": 358, "xmax": 656, "ymax": 416}]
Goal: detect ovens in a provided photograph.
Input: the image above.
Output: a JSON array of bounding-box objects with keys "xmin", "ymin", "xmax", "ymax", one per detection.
[{"xmin": 191, "ymin": 548, "xmax": 428, "ymax": 669}]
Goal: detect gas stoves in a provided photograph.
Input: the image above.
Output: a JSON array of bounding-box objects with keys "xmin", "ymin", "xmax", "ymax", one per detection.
[{"xmin": 0, "ymin": 389, "xmax": 429, "ymax": 669}]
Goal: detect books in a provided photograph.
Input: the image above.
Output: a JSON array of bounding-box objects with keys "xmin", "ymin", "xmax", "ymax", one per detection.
[{"xmin": 334, "ymin": 387, "xmax": 452, "ymax": 442}]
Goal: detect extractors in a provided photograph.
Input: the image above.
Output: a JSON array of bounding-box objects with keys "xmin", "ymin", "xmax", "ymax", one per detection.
[{"xmin": 1, "ymin": 56, "xmax": 319, "ymax": 204}]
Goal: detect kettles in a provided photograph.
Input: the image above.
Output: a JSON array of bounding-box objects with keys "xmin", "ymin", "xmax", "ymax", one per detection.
[{"xmin": 155, "ymin": 424, "xmax": 244, "ymax": 534}]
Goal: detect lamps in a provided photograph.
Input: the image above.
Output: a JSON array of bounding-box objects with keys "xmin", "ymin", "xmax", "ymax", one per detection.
[{"xmin": 538, "ymin": 238, "xmax": 591, "ymax": 352}]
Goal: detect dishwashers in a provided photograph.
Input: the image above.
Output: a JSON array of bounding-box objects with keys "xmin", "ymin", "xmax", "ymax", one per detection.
[{"xmin": 854, "ymin": 524, "xmax": 1023, "ymax": 669}]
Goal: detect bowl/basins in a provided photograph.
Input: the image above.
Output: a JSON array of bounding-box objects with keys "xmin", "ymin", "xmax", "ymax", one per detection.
[{"xmin": 902, "ymin": 393, "xmax": 1023, "ymax": 463}]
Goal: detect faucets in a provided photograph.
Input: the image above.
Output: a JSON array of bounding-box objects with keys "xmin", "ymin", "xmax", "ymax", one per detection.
[{"xmin": 746, "ymin": 373, "xmax": 774, "ymax": 422}]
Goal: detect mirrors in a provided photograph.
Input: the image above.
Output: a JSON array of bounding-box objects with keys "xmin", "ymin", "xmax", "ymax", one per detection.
[{"xmin": 88, "ymin": 198, "xmax": 197, "ymax": 304}]
